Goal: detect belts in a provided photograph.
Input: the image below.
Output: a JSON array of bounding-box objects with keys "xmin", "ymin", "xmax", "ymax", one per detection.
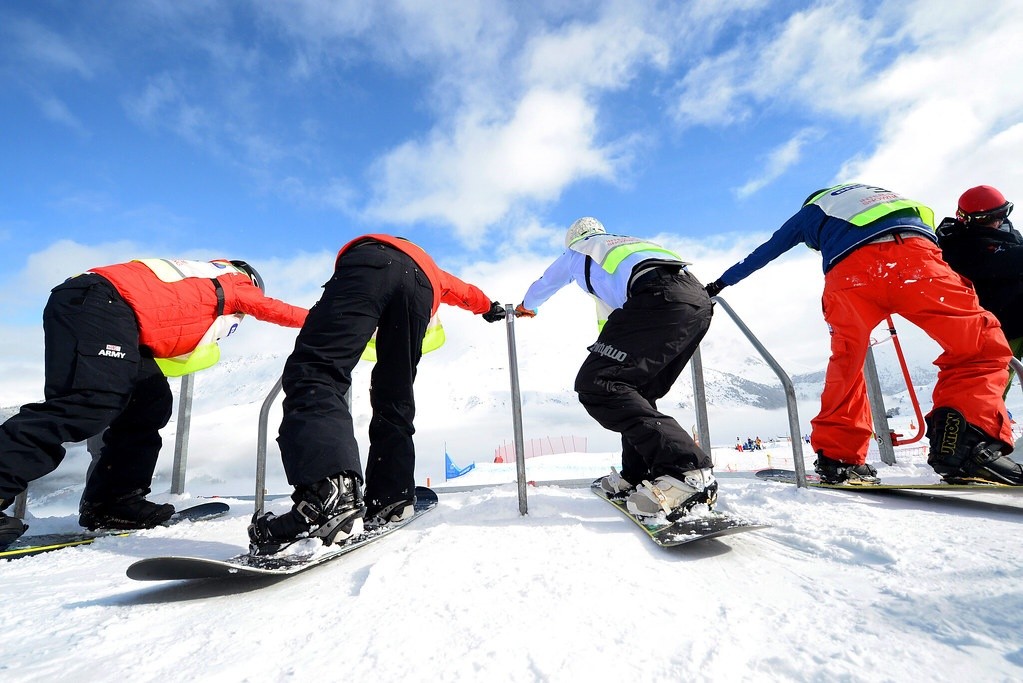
[
  {"xmin": 867, "ymin": 232, "xmax": 925, "ymax": 244},
  {"xmin": 629, "ymin": 269, "xmax": 686, "ymax": 297}
]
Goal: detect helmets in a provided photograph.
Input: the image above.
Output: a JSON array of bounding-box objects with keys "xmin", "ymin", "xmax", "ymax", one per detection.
[{"xmin": 230, "ymin": 259, "xmax": 264, "ymax": 297}]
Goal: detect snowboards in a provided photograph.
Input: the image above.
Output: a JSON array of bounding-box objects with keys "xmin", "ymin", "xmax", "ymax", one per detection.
[
  {"xmin": 591, "ymin": 472, "xmax": 776, "ymax": 551},
  {"xmin": 126, "ymin": 484, "xmax": 438, "ymax": 582},
  {"xmin": 754, "ymin": 466, "xmax": 1023, "ymax": 494},
  {"xmin": 0, "ymin": 500, "xmax": 234, "ymax": 562}
]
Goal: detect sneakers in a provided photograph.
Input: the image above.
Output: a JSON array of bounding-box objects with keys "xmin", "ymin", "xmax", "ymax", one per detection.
[
  {"xmin": 246, "ymin": 472, "xmax": 363, "ymax": 544},
  {"xmin": 927, "ymin": 407, "xmax": 1023, "ymax": 486},
  {"xmin": 0, "ymin": 511, "xmax": 30, "ymax": 552},
  {"xmin": 365, "ymin": 486, "xmax": 417, "ymax": 530},
  {"xmin": 602, "ymin": 467, "xmax": 631, "ymax": 494},
  {"xmin": 814, "ymin": 453, "xmax": 881, "ymax": 485},
  {"xmin": 627, "ymin": 469, "xmax": 719, "ymax": 518},
  {"xmin": 79, "ymin": 495, "xmax": 175, "ymax": 531}
]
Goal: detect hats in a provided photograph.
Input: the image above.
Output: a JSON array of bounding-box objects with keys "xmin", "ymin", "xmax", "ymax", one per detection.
[
  {"xmin": 957, "ymin": 184, "xmax": 1007, "ymax": 226},
  {"xmin": 565, "ymin": 216, "xmax": 606, "ymax": 248}
]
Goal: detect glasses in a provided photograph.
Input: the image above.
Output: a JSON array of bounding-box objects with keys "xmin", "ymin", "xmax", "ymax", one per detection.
[{"xmin": 975, "ymin": 201, "xmax": 1014, "ymax": 225}]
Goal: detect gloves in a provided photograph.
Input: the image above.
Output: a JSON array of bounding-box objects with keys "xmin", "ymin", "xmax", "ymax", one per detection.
[
  {"xmin": 704, "ymin": 278, "xmax": 721, "ymax": 296},
  {"xmin": 482, "ymin": 300, "xmax": 506, "ymax": 323},
  {"xmin": 514, "ymin": 301, "xmax": 535, "ymax": 319}
]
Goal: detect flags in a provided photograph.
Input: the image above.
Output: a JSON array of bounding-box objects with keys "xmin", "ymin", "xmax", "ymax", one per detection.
[{"xmin": 446, "ymin": 453, "xmax": 475, "ymax": 479}]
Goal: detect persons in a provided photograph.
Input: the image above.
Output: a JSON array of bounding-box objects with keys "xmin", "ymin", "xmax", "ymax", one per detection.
[
  {"xmin": 735, "ymin": 436, "xmax": 761, "ymax": 450},
  {"xmin": 0, "ymin": 256, "xmax": 309, "ymax": 553},
  {"xmin": 514, "ymin": 217, "xmax": 718, "ymax": 517},
  {"xmin": 805, "ymin": 433, "xmax": 809, "ymax": 444},
  {"xmin": 248, "ymin": 233, "xmax": 506, "ymax": 552},
  {"xmin": 703, "ymin": 182, "xmax": 1023, "ymax": 489},
  {"xmin": 934, "ymin": 184, "xmax": 1023, "ymax": 424}
]
